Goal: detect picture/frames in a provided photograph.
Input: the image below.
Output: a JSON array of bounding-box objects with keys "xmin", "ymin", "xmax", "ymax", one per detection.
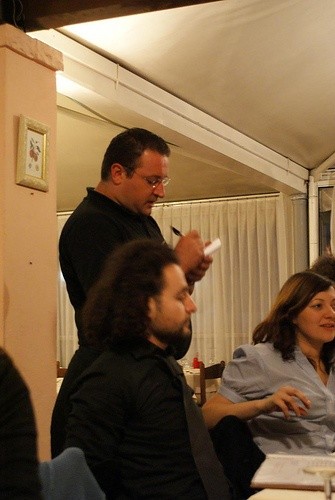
[{"xmin": 15, "ymin": 113, "xmax": 50, "ymax": 194}]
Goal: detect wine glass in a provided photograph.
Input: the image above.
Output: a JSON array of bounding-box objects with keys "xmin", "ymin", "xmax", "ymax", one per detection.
[{"xmin": 302, "ymin": 464, "xmax": 335, "ymax": 500}]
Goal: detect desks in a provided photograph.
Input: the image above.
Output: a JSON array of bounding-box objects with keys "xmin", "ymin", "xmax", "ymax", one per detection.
[{"xmin": 185, "ymin": 369, "xmax": 221, "ymax": 403}]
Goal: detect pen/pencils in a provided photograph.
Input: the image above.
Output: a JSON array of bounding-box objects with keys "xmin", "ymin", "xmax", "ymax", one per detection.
[{"xmin": 170, "ymin": 225, "xmax": 184, "ymax": 238}]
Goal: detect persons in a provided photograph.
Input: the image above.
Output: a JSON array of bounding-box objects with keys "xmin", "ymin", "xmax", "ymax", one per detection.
[
  {"xmin": 200, "ymin": 270, "xmax": 335, "ymax": 456},
  {"xmin": 59, "ymin": 127, "xmax": 212, "ymax": 360},
  {"xmin": 309, "ymin": 254, "xmax": 335, "ymax": 282},
  {"xmin": 50, "ymin": 237, "xmax": 231, "ymax": 500},
  {"xmin": 0, "ymin": 345, "xmax": 42, "ymax": 500}
]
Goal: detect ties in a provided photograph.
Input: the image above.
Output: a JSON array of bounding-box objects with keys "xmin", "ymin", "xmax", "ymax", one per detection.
[{"xmin": 155, "ymin": 355, "xmax": 231, "ymax": 500}]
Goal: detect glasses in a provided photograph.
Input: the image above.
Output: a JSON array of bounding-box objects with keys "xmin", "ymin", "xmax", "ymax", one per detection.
[{"xmin": 124, "ymin": 165, "xmax": 168, "ymax": 187}]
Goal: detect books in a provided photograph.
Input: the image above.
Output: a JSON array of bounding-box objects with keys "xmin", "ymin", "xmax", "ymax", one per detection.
[
  {"xmin": 251, "ymin": 453, "xmax": 335, "ymax": 491},
  {"xmin": 203, "ymin": 239, "xmax": 221, "ymax": 255}
]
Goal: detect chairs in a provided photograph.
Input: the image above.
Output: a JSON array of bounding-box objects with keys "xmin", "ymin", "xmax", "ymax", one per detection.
[{"xmin": 200, "ymin": 359, "xmax": 225, "ymax": 405}]
